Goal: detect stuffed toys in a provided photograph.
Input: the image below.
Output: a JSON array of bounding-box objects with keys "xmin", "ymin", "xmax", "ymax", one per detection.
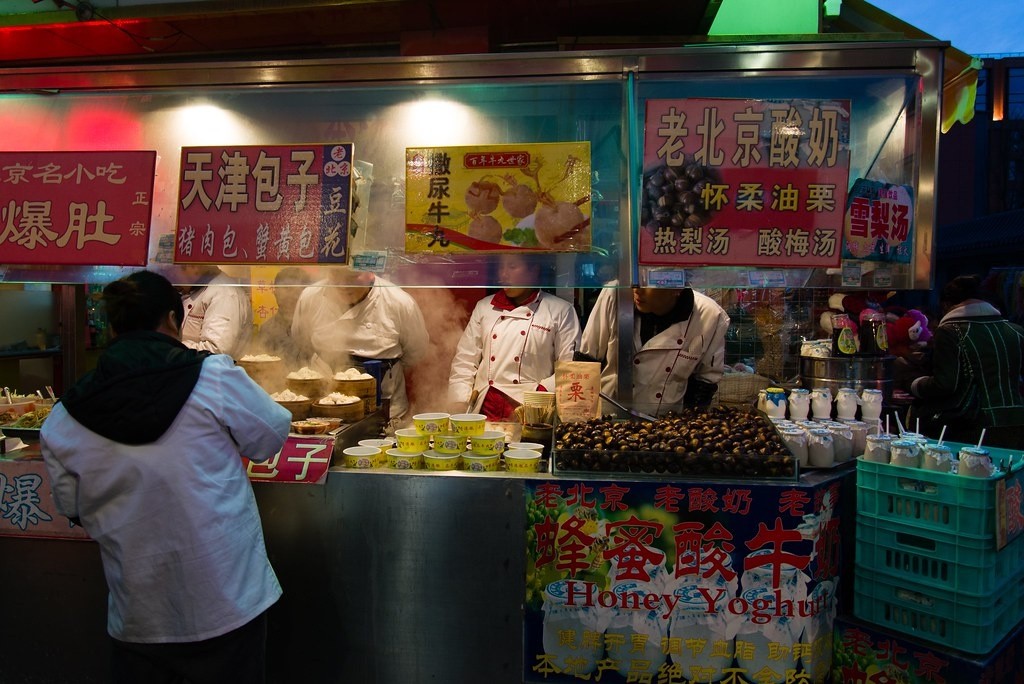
[{"xmin": 820, "ymin": 292, "xmax": 933, "ymax": 365}]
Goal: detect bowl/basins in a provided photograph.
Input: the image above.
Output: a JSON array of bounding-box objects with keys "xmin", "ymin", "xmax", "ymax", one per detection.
[
  {"xmin": 395, "ymin": 428, "xmax": 430, "ymax": 453},
  {"xmin": 461, "ymin": 451, "xmax": 501, "ymax": 471},
  {"xmin": 423, "ymin": 450, "xmax": 460, "ymax": 470},
  {"xmin": 412, "ymin": 413, "xmax": 451, "ymax": 434},
  {"xmin": 385, "ymin": 448, "xmax": 423, "ymax": 469},
  {"xmin": 291, "ymin": 421, "xmax": 330, "ymax": 436},
  {"xmin": 342, "ymin": 446, "xmax": 382, "ymax": 468},
  {"xmin": 0, "ymin": 396, "xmax": 36, "ymax": 418},
  {"xmin": 433, "ymin": 429, "xmax": 467, "ymax": 453},
  {"xmin": 470, "ymin": 430, "xmax": 506, "ymax": 454},
  {"xmin": 35, "ymin": 398, "xmax": 60, "ymax": 409},
  {"xmin": 503, "ymin": 450, "xmax": 542, "ymax": 473},
  {"xmin": 507, "ymin": 443, "xmax": 544, "ymax": 454},
  {"xmin": 306, "ymin": 417, "xmax": 342, "ymax": 430},
  {"xmin": 449, "ymin": 414, "xmax": 487, "ymax": 435},
  {"xmin": 523, "ymin": 391, "xmax": 556, "ymax": 406},
  {"xmin": 358, "ymin": 439, "xmax": 393, "ymax": 461}
]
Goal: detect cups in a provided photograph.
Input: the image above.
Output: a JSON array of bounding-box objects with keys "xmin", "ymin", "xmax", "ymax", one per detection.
[
  {"xmin": 800, "ymin": 339, "xmax": 819, "ymax": 356},
  {"xmin": 520, "ymin": 423, "xmax": 553, "ymax": 458},
  {"xmin": 812, "ymin": 344, "xmax": 830, "ymax": 357},
  {"xmin": 817, "ymin": 339, "xmax": 832, "ymax": 350}
]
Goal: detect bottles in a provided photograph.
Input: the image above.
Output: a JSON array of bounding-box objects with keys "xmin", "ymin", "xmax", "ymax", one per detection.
[
  {"xmin": 87, "ymin": 284, "xmax": 107, "ymax": 348},
  {"xmin": 811, "ymin": 388, "xmax": 832, "ymax": 419},
  {"xmin": 830, "ymin": 314, "xmax": 858, "ymax": 357},
  {"xmin": 861, "ymin": 389, "xmax": 882, "ymax": 420},
  {"xmin": 765, "ymin": 387, "xmax": 787, "ymax": 419},
  {"xmin": 37, "ymin": 327, "xmax": 46, "ymax": 349},
  {"xmin": 771, "ymin": 419, "xmax": 866, "ymax": 467},
  {"xmin": 788, "ymin": 389, "xmax": 810, "ymax": 420},
  {"xmin": 757, "ymin": 389, "xmax": 766, "ymax": 412},
  {"xmin": 859, "ymin": 313, "xmax": 889, "ymax": 356},
  {"xmin": 837, "ymin": 388, "xmax": 857, "ymax": 420},
  {"xmin": 865, "ymin": 433, "xmax": 990, "ymax": 523}
]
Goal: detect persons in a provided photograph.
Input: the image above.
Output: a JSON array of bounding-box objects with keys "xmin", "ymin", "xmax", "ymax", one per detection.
[
  {"xmin": 893, "ymin": 273, "xmax": 1024, "ymax": 451},
  {"xmin": 151, "ymin": 258, "xmax": 253, "ymax": 376},
  {"xmin": 579, "ymin": 260, "xmax": 730, "ymax": 427},
  {"xmin": 291, "ymin": 260, "xmax": 437, "ymax": 437},
  {"xmin": 38, "ymin": 270, "xmax": 294, "ymax": 684},
  {"xmin": 256, "ymin": 268, "xmax": 319, "ymax": 381},
  {"xmin": 446, "ymin": 252, "xmax": 581, "ymax": 428}
]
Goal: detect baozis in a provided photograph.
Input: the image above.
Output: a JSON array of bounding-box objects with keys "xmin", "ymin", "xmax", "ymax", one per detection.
[{"xmin": 241, "ymin": 354, "xmax": 373, "ymax": 405}]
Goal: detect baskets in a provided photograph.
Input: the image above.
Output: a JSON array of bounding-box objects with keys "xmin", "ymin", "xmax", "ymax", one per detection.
[{"xmin": 718, "ymin": 373, "xmax": 772, "ymax": 403}]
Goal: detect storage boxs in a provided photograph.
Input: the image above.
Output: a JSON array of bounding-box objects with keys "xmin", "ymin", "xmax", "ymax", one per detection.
[{"xmin": 854, "ymin": 437, "xmax": 1024, "ymax": 655}]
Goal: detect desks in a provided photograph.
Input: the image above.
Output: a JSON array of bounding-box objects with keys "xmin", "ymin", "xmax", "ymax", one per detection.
[{"xmin": 0, "ymin": 437, "xmax": 855, "ymax": 684}]
